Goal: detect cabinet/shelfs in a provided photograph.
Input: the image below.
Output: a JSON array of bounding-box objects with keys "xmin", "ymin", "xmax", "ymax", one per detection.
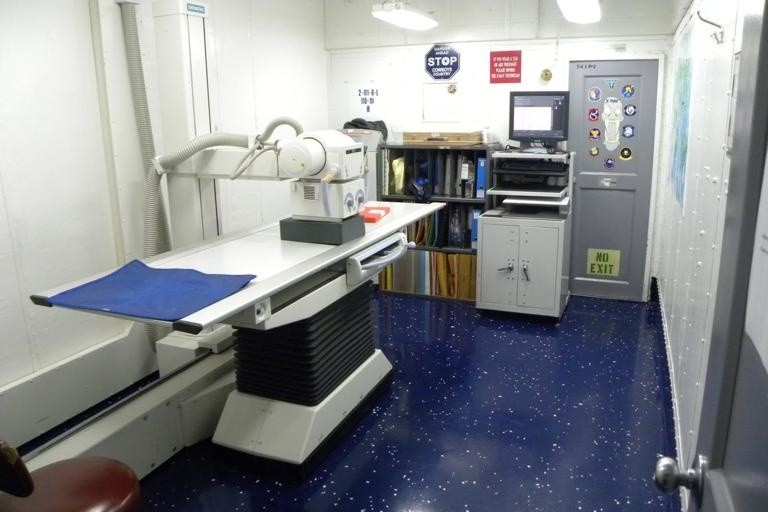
[{"xmin": 377, "ymin": 141, "xmax": 577, "ymax": 324}]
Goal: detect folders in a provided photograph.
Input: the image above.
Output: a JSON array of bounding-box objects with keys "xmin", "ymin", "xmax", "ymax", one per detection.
[
  {"xmin": 471, "ymin": 209, "xmax": 482, "ymax": 251},
  {"xmin": 433, "ymin": 152, "xmax": 486, "ymax": 200}
]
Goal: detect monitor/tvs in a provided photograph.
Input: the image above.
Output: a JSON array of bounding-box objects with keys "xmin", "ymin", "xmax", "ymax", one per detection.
[{"xmin": 508, "ymin": 91, "xmax": 570, "ymax": 154}]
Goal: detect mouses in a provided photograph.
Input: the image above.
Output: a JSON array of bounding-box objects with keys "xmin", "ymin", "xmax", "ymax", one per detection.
[{"xmin": 547, "ymin": 146, "xmax": 555, "ymax": 154}]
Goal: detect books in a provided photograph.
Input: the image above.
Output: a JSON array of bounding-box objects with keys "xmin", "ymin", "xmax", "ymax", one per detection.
[
  {"xmin": 359, "ymin": 206, "xmax": 389, "ymax": 223},
  {"xmin": 374, "ymin": 150, "xmax": 485, "ymax": 304},
  {"xmin": 377, "ymin": 295, "xmax": 474, "ymax": 352}
]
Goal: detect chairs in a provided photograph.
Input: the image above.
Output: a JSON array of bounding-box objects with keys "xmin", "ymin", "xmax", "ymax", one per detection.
[{"xmin": 0, "ymin": 439, "xmax": 142, "ymax": 512}]
